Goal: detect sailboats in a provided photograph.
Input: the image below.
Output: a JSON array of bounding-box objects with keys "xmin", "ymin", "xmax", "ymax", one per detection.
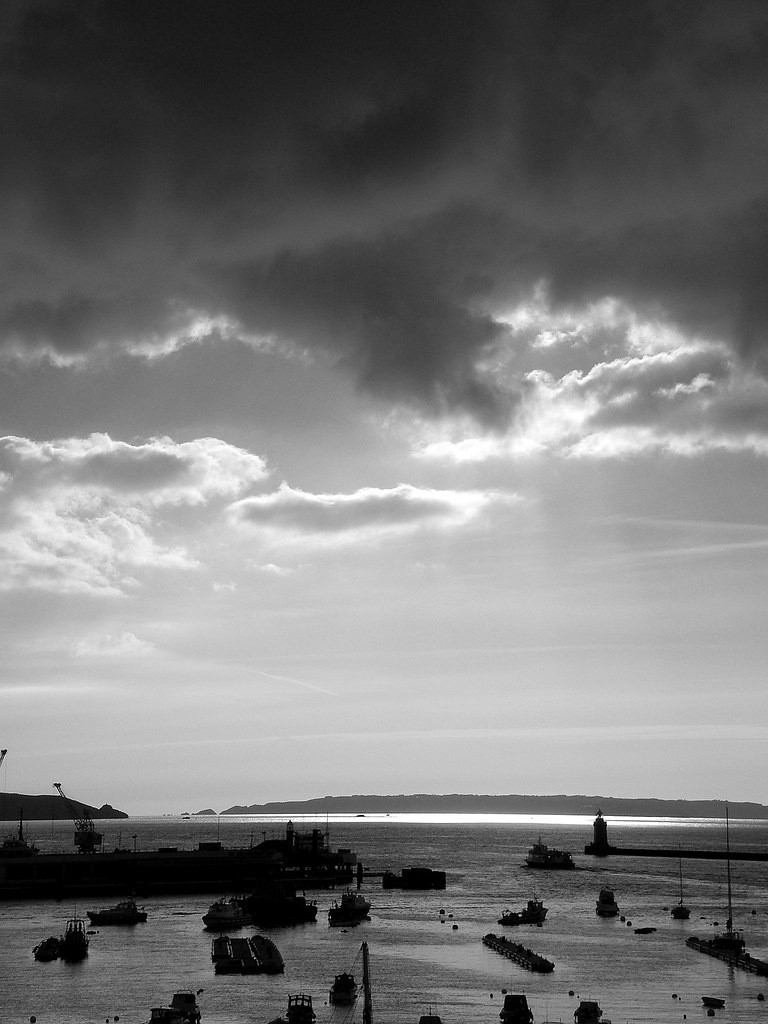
[{"xmin": 671, "ymin": 843, "xmax": 693, "ymax": 919}]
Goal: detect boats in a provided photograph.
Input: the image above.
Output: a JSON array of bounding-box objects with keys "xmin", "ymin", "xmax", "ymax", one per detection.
[
  {"xmin": 325, "ymin": 969, "xmax": 356, "ymax": 1007},
  {"xmin": 499, "ymin": 894, "xmax": 549, "ymax": 925},
  {"xmin": 324, "ymin": 885, "xmax": 373, "ymax": 927},
  {"xmin": 199, "ymin": 896, "xmax": 248, "ymax": 929},
  {"xmin": 143, "ymin": 987, "xmax": 730, "ymax": 1024},
  {"xmin": 523, "ymin": 836, "xmax": 579, "ymax": 872},
  {"xmin": 594, "ymin": 885, "xmax": 620, "ymax": 917},
  {"xmin": 33, "ymin": 933, "xmax": 63, "ymax": 962},
  {"xmin": 81, "ymin": 899, "xmax": 153, "ymax": 925},
  {"xmin": 60, "ymin": 913, "xmax": 92, "ymax": 966}
]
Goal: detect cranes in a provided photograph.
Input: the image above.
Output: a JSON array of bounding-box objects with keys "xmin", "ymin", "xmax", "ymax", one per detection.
[{"xmin": 52, "ymin": 781, "xmax": 104, "ymax": 853}]
